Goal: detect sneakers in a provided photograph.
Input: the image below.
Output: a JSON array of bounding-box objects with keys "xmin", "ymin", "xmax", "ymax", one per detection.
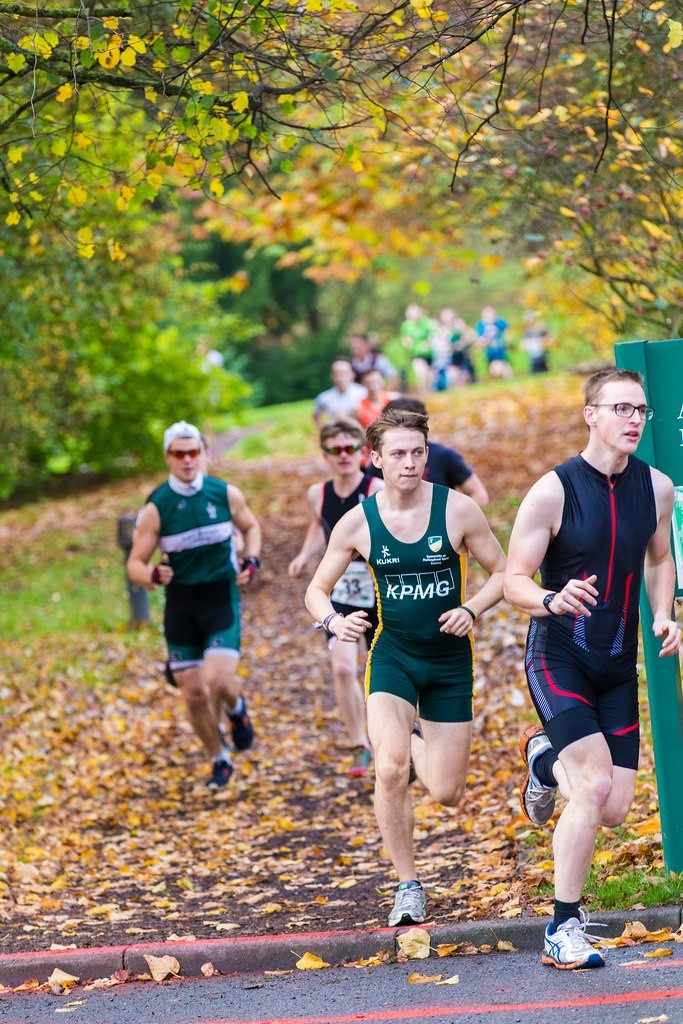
[
  {"xmin": 541, "ymin": 906, "xmax": 608, "ymax": 969},
  {"xmin": 408, "ymin": 721, "xmax": 424, "ymax": 785},
  {"xmin": 205, "ymin": 756, "xmax": 236, "ymax": 792},
  {"xmin": 348, "ymin": 743, "xmax": 374, "ymax": 777},
  {"xmin": 388, "ymin": 885, "xmax": 427, "ymax": 927},
  {"xmin": 224, "ymin": 692, "xmax": 255, "ymax": 751},
  {"xmin": 520, "ymin": 724, "xmax": 559, "ymax": 825}
]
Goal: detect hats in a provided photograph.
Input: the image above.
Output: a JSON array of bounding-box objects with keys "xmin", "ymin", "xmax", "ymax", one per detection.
[{"xmin": 163, "ymin": 420, "xmax": 201, "ymax": 452}]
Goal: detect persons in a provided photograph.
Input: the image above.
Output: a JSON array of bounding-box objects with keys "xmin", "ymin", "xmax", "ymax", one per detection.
[
  {"xmin": 125, "ymin": 418, "xmax": 263, "ymax": 792},
  {"xmin": 503, "ymin": 369, "xmax": 682, "ymax": 970},
  {"xmin": 304, "ymin": 409, "xmax": 507, "ymax": 926},
  {"xmin": 288, "ymin": 416, "xmax": 387, "ymax": 779},
  {"xmin": 365, "ymin": 397, "xmax": 489, "ymax": 511},
  {"xmin": 308, "ymin": 300, "xmax": 550, "ymax": 473}
]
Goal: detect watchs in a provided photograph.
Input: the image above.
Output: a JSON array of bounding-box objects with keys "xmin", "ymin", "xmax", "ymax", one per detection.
[{"xmin": 542, "ymin": 592, "xmax": 558, "ymax": 618}]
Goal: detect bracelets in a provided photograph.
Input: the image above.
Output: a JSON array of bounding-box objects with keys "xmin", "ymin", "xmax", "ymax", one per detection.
[
  {"xmin": 313, "ymin": 611, "xmax": 344, "ymax": 632},
  {"xmin": 253, "ymin": 556, "xmax": 261, "ymax": 567},
  {"xmin": 459, "ymin": 604, "xmax": 476, "ymax": 622}
]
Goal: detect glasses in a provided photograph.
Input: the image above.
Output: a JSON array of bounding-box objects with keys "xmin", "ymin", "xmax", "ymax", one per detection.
[
  {"xmin": 321, "ymin": 445, "xmax": 362, "ymax": 456},
  {"xmin": 168, "ymin": 448, "xmax": 201, "ymax": 459},
  {"xmin": 590, "ymin": 403, "xmax": 655, "ymax": 421}
]
